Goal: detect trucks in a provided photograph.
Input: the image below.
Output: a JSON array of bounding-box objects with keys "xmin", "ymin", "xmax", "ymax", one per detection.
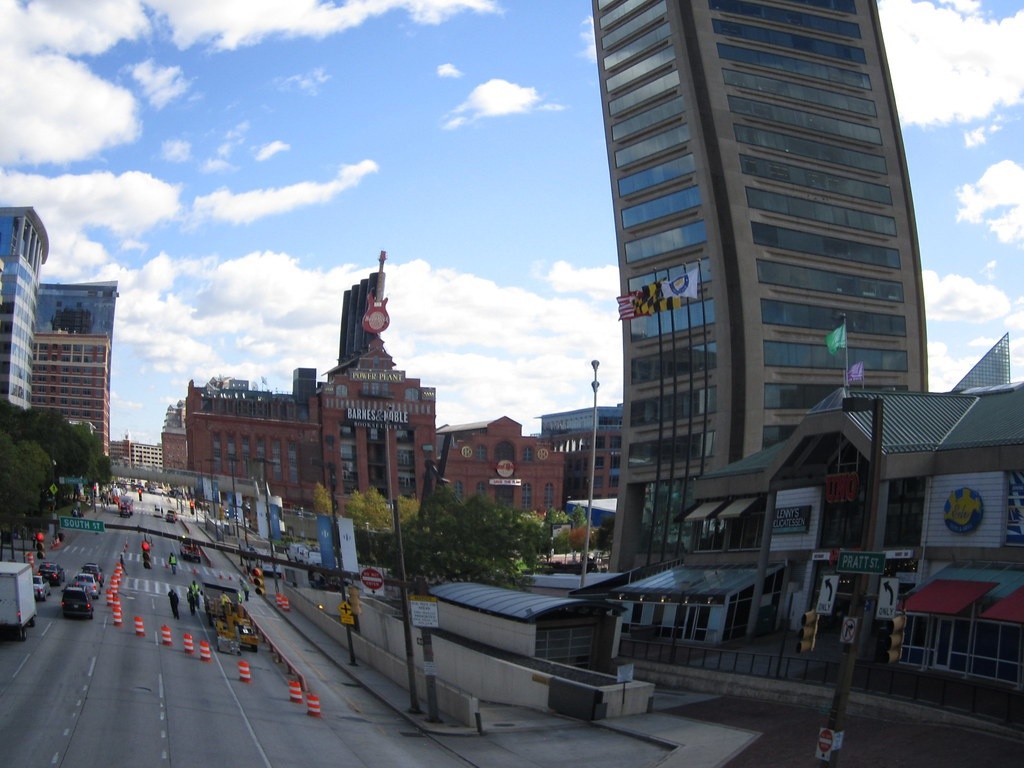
[
  {"xmin": 0, "ymin": 561, "xmax": 38, "ymax": 642},
  {"xmin": 118, "ymin": 495, "xmax": 134, "ymax": 519},
  {"xmin": 197, "ymin": 581, "xmax": 259, "ymax": 653}
]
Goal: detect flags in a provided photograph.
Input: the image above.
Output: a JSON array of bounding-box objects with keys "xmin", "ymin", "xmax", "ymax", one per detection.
[
  {"xmin": 825, "ymin": 324, "xmax": 847, "ymax": 354},
  {"xmin": 844, "ymin": 363, "xmax": 865, "ymax": 384},
  {"xmin": 618, "ymin": 268, "xmax": 698, "ymax": 321}
]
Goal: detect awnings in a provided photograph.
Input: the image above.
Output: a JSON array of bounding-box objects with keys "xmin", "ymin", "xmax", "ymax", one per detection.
[{"xmin": 673, "ymin": 498, "xmax": 757, "ymax": 522}]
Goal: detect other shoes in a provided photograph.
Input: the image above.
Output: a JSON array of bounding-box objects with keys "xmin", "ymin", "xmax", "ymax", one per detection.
[
  {"xmin": 196, "ymin": 605, "xmax": 200, "ymax": 609},
  {"xmin": 173, "ymin": 616, "xmax": 179, "ymax": 619},
  {"xmin": 245, "ymin": 598, "xmax": 250, "ymax": 601},
  {"xmin": 192, "ymin": 613, "xmax": 196, "ymax": 616}
]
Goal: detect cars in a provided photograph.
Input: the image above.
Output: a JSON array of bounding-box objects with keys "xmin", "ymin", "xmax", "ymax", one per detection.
[
  {"xmin": 38, "ymin": 561, "xmax": 66, "ymax": 586},
  {"xmin": 33, "ymin": 575, "xmax": 52, "ymax": 602},
  {"xmin": 72, "ymin": 574, "xmax": 102, "ymax": 599},
  {"xmin": 81, "ymin": 563, "xmax": 105, "ymax": 587},
  {"xmin": 60, "ymin": 584, "xmax": 94, "ymax": 620},
  {"xmin": 180, "ymin": 534, "xmax": 201, "ymax": 563},
  {"xmin": 152, "ymin": 503, "xmax": 163, "ymax": 519},
  {"xmin": 166, "ymin": 509, "xmax": 177, "ymax": 524}
]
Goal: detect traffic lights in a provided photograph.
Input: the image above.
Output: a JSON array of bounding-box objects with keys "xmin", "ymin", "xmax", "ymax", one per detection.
[
  {"xmin": 252, "ymin": 567, "xmax": 266, "ymax": 597},
  {"xmin": 190, "ymin": 501, "xmax": 195, "ymax": 515},
  {"xmin": 37, "ymin": 532, "xmax": 46, "ymax": 561},
  {"xmin": 138, "ymin": 489, "xmax": 143, "ymax": 502},
  {"xmin": 141, "ymin": 540, "xmax": 153, "ymax": 570},
  {"xmin": 94, "ymin": 486, "xmax": 97, "ymax": 497}
]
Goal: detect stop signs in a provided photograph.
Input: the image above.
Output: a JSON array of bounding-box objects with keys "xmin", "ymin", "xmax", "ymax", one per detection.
[{"xmin": 819, "ymin": 730, "xmax": 833, "ymax": 752}]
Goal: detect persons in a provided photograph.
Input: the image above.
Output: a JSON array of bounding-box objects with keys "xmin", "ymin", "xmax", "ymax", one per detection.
[{"xmin": 33, "ymin": 489, "xmax": 251, "ymax": 619}]
